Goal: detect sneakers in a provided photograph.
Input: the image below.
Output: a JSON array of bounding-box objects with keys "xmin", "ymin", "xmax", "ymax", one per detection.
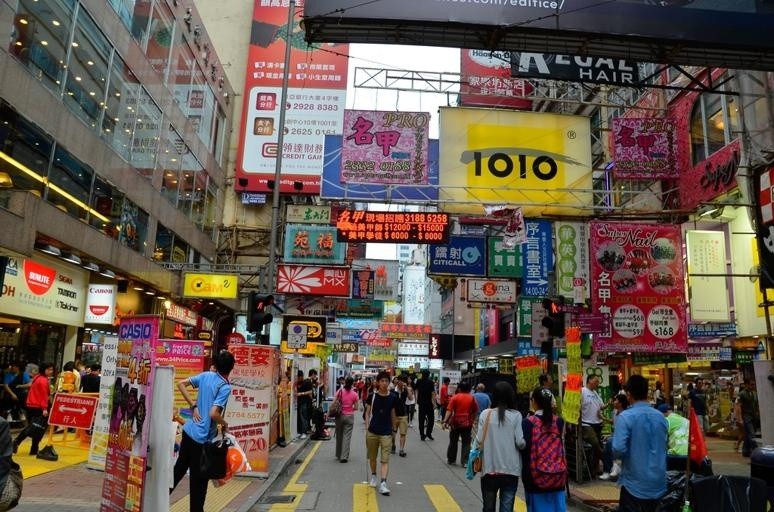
[
  {"xmin": 399, "ymin": 450, "xmax": 406, "ymax": 457},
  {"xmin": 421, "ymin": 435, "xmax": 426, "ymax": 440},
  {"xmin": 391, "ymin": 446, "xmax": 395, "ymax": 454},
  {"xmin": 370, "ymin": 475, "xmax": 377, "ymax": 487},
  {"xmin": 599, "ymin": 472, "xmax": 610, "ymax": 479},
  {"xmin": 379, "ymin": 482, "xmax": 390, "ymax": 493},
  {"xmin": 610, "ymin": 464, "xmax": 621, "ymax": 476},
  {"xmin": 427, "ymin": 434, "xmax": 433, "ymax": 440}
]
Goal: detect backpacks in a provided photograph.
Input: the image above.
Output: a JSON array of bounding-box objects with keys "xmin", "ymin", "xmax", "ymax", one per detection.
[{"xmin": 527, "ymin": 416, "xmax": 568, "ymax": 491}]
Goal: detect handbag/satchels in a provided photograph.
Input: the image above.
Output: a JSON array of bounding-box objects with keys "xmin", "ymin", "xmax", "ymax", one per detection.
[
  {"xmin": 328, "ymin": 399, "xmax": 341, "ymax": 417},
  {"xmin": 473, "ymin": 457, "xmax": 481, "ymax": 473},
  {"xmin": 26, "ymin": 415, "xmax": 47, "ymax": 440},
  {"xmin": 194, "ymin": 440, "xmax": 226, "ymax": 479},
  {"xmin": 0, "ymin": 458, "xmax": 23, "ymax": 511},
  {"xmin": 466, "ymin": 450, "xmax": 479, "ymax": 480}
]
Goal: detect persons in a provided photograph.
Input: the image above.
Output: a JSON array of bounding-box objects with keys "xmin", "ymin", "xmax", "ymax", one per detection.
[
  {"xmin": 1, "ymin": 358, "xmax": 101, "ymax": 454},
  {"xmin": 169, "ymin": 352, "xmax": 235, "ymax": 512},
  {"xmin": 297, "ymin": 359, "xmax": 773, "ymax": 512}
]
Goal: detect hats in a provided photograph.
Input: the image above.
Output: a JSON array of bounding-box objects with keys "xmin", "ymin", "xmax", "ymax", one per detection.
[{"xmin": 658, "ymin": 404, "xmax": 671, "ymax": 410}]
